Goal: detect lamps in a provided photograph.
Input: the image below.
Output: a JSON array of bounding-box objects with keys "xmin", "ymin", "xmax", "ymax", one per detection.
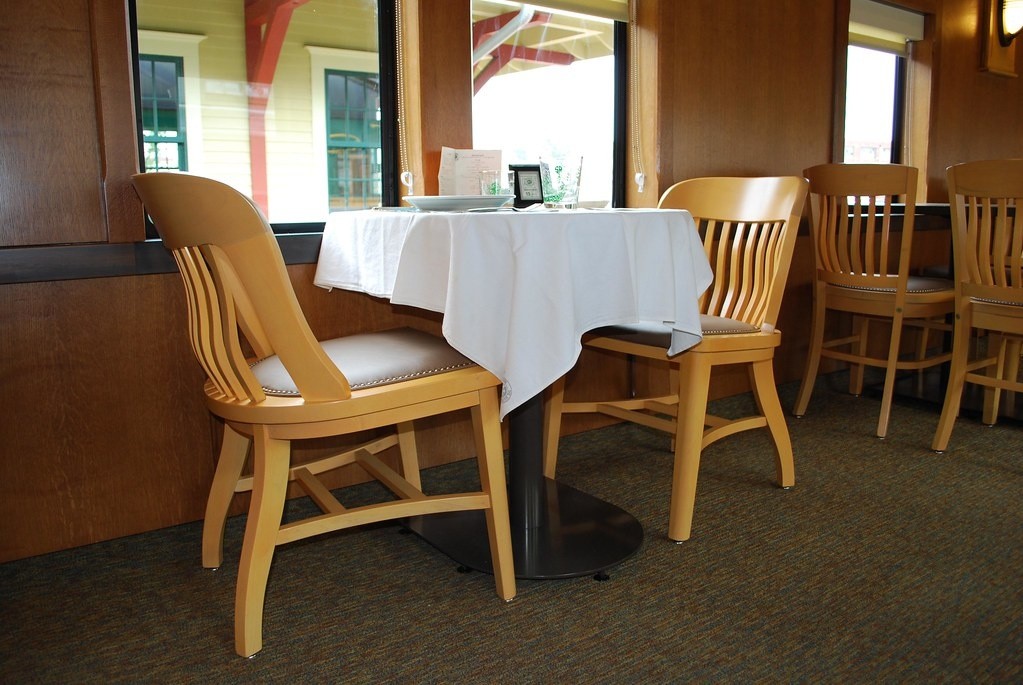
[{"xmin": 997, "ymin": 0, "xmax": 1023, "ymax": 48}]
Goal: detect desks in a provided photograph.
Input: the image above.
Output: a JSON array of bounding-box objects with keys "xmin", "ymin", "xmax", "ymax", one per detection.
[
  {"xmin": 833, "ymin": 202, "xmax": 1016, "ymax": 419},
  {"xmin": 311, "ymin": 206, "xmax": 717, "ymax": 580}
]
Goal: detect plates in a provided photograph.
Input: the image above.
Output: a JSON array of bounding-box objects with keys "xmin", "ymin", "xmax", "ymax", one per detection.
[{"xmin": 402, "ymin": 195, "xmax": 513, "ymax": 213}]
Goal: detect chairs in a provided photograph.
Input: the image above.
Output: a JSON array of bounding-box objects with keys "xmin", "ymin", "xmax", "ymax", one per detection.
[
  {"xmin": 130, "ymin": 170, "xmax": 516, "ymax": 661},
  {"xmin": 791, "ymin": 162, "xmax": 955, "ymax": 441},
  {"xmin": 541, "ymin": 176, "xmax": 810, "ymax": 546},
  {"xmin": 929, "ymin": 158, "xmax": 1023, "ymax": 457}
]
